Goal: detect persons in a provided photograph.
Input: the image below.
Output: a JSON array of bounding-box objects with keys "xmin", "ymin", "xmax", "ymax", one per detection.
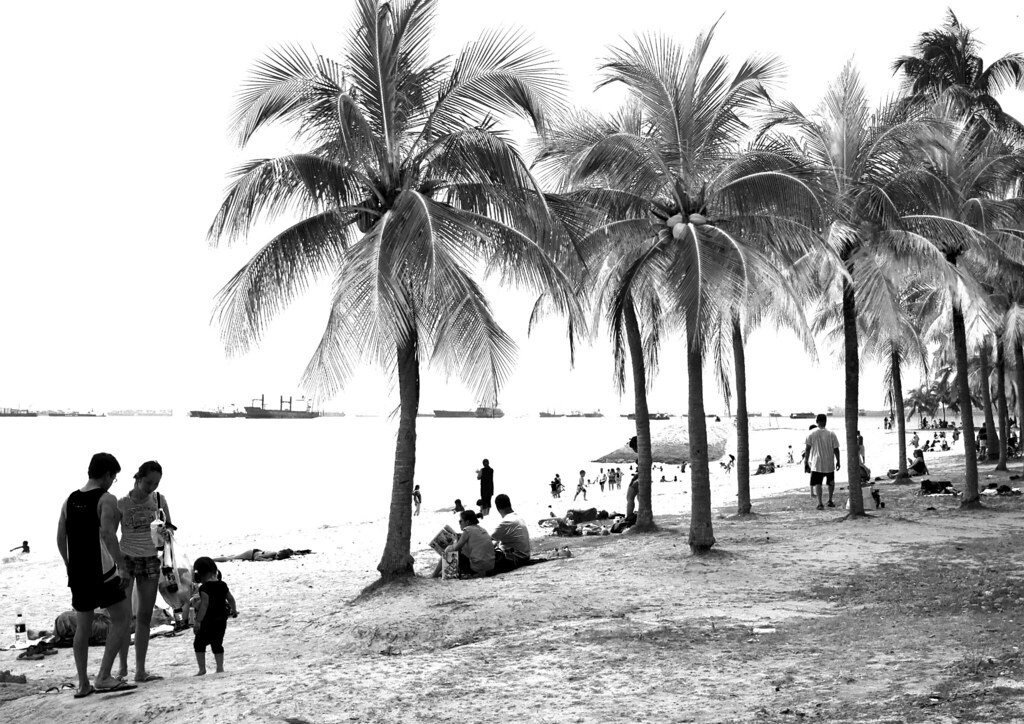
[
  {"xmin": 114, "ymin": 461, "xmax": 176, "ymax": 681},
  {"xmin": 189, "ymin": 556, "xmax": 238, "ymax": 677},
  {"xmin": 979, "ymin": 418, "xmax": 1019, "ymax": 460},
  {"xmin": 858, "ymin": 413, "xmax": 961, "ymax": 479},
  {"xmin": 549, "ymin": 435, "xmax": 640, "ymax": 518},
  {"xmin": 26, "ymin": 611, "xmax": 168, "ymax": 642},
  {"xmin": 412, "ymin": 485, "xmax": 422, "ymax": 516},
  {"xmin": 9, "ymin": 540, "xmax": 31, "ymax": 554},
  {"xmin": 804, "ymin": 414, "xmax": 840, "ymax": 510},
  {"xmin": 213, "ymin": 549, "xmax": 292, "ymax": 562},
  {"xmin": 429, "ymin": 459, "xmax": 531, "ymax": 584},
  {"xmin": 57, "ymin": 453, "xmax": 140, "ymax": 696},
  {"xmin": 652, "ymin": 425, "xmax": 817, "ymax": 498}
]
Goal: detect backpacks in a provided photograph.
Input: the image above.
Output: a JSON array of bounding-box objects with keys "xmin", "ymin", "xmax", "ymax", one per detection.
[{"xmin": 554, "ymin": 507, "xmax": 637, "ymax": 537}]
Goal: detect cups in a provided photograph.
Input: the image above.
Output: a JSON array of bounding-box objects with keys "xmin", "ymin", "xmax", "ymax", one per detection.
[{"xmin": 150, "ymin": 520, "xmax": 166, "ymax": 548}]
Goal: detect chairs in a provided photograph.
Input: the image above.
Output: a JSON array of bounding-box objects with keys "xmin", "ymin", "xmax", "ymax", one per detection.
[{"xmin": 1007, "ymin": 443, "xmax": 1023, "ymax": 461}]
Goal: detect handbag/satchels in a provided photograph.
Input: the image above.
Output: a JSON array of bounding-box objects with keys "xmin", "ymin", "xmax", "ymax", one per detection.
[
  {"xmin": 158, "ymin": 530, "xmax": 193, "ymax": 608},
  {"xmin": 846, "ymin": 487, "xmax": 877, "ymax": 510}
]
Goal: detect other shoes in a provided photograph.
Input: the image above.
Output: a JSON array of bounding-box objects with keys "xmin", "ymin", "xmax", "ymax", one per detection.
[{"xmin": 816, "ymin": 500, "xmax": 835, "ymax": 510}]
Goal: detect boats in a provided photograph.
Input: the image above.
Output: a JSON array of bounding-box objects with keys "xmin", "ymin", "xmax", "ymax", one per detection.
[
  {"xmin": 627, "ymin": 413, "xmax": 669, "ymax": 420},
  {"xmin": 246, "ymin": 407, "xmax": 321, "ymax": 417},
  {"xmin": 433, "ymin": 407, "xmax": 505, "ymax": 418},
  {"xmin": 789, "ymin": 413, "xmax": 816, "ymax": 419},
  {"xmin": 538, "ymin": 411, "xmax": 604, "ymax": 417}
]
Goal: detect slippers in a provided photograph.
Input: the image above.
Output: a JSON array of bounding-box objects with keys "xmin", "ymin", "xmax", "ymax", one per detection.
[
  {"xmin": 74, "ymin": 682, "xmax": 137, "ymax": 697},
  {"xmin": 135, "ymin": 674, "xmax": 164, "ymax": 682},
  {"xmin": 17, "ymin": 641, "xmax": 57, "ymax": 660},
  {"xmin": 115, "ymin": 670, "xmax": 128, "ymax": 679}
]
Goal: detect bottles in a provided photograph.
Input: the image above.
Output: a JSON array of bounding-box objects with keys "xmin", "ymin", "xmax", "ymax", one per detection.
[{"xmin": 14, "ymin": 614, "xmax": 26, "ymax": 649}]
